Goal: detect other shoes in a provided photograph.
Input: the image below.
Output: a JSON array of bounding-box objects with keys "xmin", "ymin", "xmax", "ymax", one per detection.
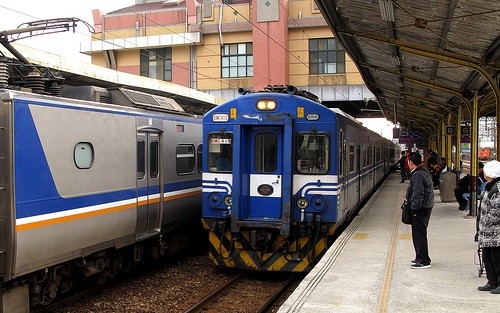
[
  {"xmin": 478, "ymin": 283, "xmax": 498, "ymax": 291},
  {"xmin": 459, "ymin": 199, "xmax": 468, "ymax": 211},
  {"xmin": 490, "ymin": 286, "xmax": 500, "ymax": 294}
]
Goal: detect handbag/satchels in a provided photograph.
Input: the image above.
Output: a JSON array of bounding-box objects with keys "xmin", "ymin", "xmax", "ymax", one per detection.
[{"xmin": 401, "ymin": 201, "xmax": 412, "ymax": 224}]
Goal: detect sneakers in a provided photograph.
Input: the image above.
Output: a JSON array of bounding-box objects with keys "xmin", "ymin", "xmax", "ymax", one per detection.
[
  {"xmin": 410, "ymin": 262, "xmax": 432, "ymax": 269},
  {"xmin": 411, "ymin": 259, "xmax": 431, "ymax": 264}
]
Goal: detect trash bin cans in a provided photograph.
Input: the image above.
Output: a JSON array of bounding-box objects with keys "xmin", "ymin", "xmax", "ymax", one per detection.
[{"xmin": 438, "ymin": 172, "xmax": 457, "ymax": 202}]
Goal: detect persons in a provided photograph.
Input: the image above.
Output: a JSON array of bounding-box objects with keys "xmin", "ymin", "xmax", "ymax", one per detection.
[
  {"xmin": 406, "ymin": 152, "xmax": 434, "ymax": 269},
  {"xmin": 432, "ymin": 157, "xmax": 446, "ymax": 187},
  {"xmin": 427, "ymin": 149, "xmax": 438, "ymax": 173},
  {"xmin": 394, "ymin": 151, "xmax": 407, "ymax": 183},
  {"xmin": 214, "ymin": 145, "xmax": 232, "ymax": 170},
  {"xmin": 454, "ymin": 162, "xmax": 487, "ymax": 211},
  {"xmin": 474, "ymin": 160, "xmax": 500, "ymax": 294}
]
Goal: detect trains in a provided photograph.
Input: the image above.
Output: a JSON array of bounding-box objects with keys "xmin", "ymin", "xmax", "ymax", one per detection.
[
  {"xmin": 0, "ymin": 16, "xmax": 231, "ymax": 313},
  {"xmin": 200, "ymin": 85, "xmax": 405, "ymax": 274}
]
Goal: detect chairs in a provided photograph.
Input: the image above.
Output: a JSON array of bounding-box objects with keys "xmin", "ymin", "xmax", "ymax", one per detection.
[{"xmin": 463, "ymin": 182, "xmax": 489, "ymax": 208}]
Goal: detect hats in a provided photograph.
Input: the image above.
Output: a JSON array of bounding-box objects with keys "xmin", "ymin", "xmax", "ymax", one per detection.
[
  {"xmin": 482, "ymin": 160, "xmax": 500, "ymax": 178},
  {"xmin": 428, "ymin": 149, "xmax": 433, "ymax": 154},
  {"xmin": 401, "ymin": 151, "xmax": 405, "ymax": 154}
]
